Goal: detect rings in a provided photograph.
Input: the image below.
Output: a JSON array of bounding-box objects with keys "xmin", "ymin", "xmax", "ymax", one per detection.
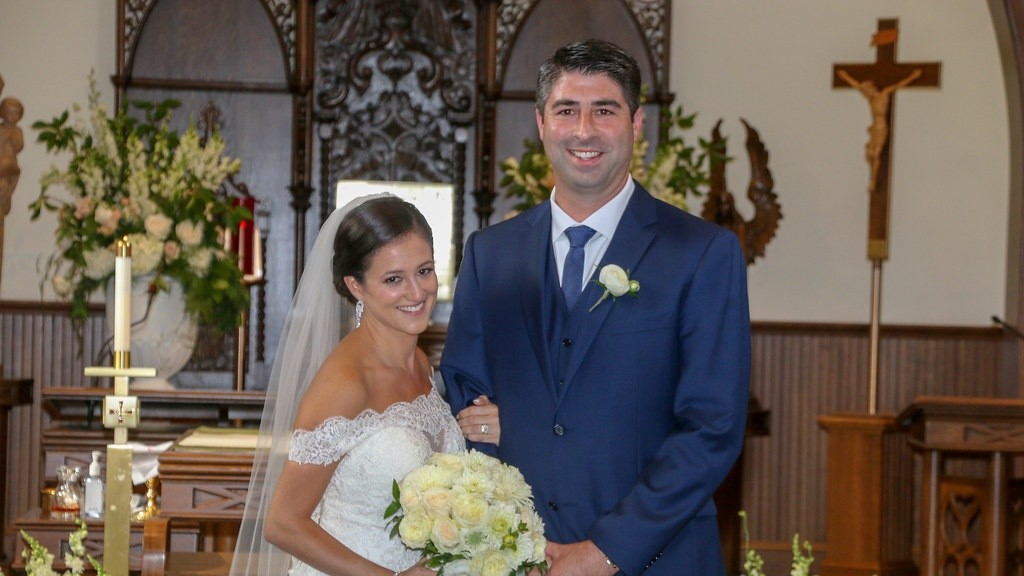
[{"xmin": 481, "ymin": 423, "xmax": 488, "ymax": 434}]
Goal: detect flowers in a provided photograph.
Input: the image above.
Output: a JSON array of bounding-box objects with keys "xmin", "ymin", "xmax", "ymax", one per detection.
[
  {"xmin": 497, "ymin": 79, "xmax": 736, "ymax": 225},
  {"xmin": 380, "ymin": 448, "xmax": 548, "ymax": 576},
  {"xmin": 586, "ymin": 263, "xmax": 640, "ymax": 313},
  {"xmin": 23, "ymin": 67, "xmax": 265, "ymax": 336}
]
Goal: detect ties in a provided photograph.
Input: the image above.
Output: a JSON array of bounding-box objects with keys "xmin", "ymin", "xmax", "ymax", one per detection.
[{"xmin": 563, "ymin": 225, "xmax": 597, "ymax": 311}]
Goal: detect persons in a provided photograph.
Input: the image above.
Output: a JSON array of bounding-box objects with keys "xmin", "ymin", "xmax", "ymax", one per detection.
[
  {"xmin": 435, "ymin": 40, "xmax": 751, "ymax": 576},
  {"xmin": 838, "ymin": 68, "xmax": 922, "ymax": 194},
  {"xmin": 0, "ymin": 97, "xmax": 24, "ymax": 217},
  {"xmin": 227, "ymin": 190, "xmax": 503, "ymax": 576}
]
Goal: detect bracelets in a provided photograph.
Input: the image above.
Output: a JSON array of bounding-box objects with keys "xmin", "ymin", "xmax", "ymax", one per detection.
[
  {"xmin": 393, "ymin": 572, "xmax": 400, "ymax": 576},
  {"xmin": 597, "ymin": 546, "xmax": 617, "ymax": 570}
]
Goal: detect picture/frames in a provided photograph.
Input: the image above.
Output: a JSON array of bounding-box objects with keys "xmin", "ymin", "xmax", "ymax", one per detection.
[{"xmin": 103, "ymin": 266, "xmax": 200, "ymax": 391}]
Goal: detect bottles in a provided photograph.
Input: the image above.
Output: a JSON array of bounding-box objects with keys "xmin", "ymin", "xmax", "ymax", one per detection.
[{"xmin": 49, "ymin": 466, "xmax": 82, "ymax": 522}]
